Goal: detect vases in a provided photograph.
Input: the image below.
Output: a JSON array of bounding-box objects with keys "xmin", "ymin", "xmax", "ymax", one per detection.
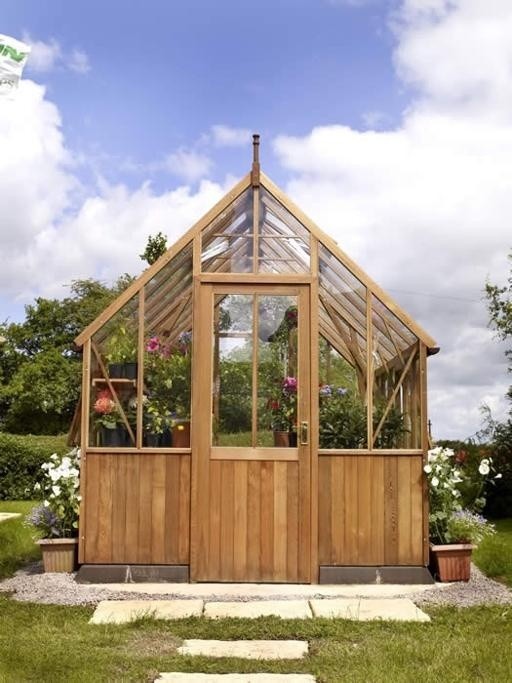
[
  {"xmin": 102, "ymin": 422, "xmax": 190, "ymax": 447},
  {"xmin": 428, "ymin": 544, "xmax": 476, "ymax": 583},
  {"xmin": 272, "ymin": 432, "xmax": 294, "ymax": 446},
  {"xmin": 34, "ymin": 538, "xmax": 78, "ymax": 573}
]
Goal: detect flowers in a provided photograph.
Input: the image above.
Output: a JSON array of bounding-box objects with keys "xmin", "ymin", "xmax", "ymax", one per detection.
[
  {"xmin": 88, "ymin": 311, "xmax": 193, "ymax": 434},
  {"xmin": 422, "ymin": 445, "xmax": 502, "ymax": 544},
  {"xmin": 19, "ymin": 445, "xmax": 79, "ymax": 536},
  {"xmin": 266, "ymin": 377, "xmax": 349, "ymax": 433}
]
